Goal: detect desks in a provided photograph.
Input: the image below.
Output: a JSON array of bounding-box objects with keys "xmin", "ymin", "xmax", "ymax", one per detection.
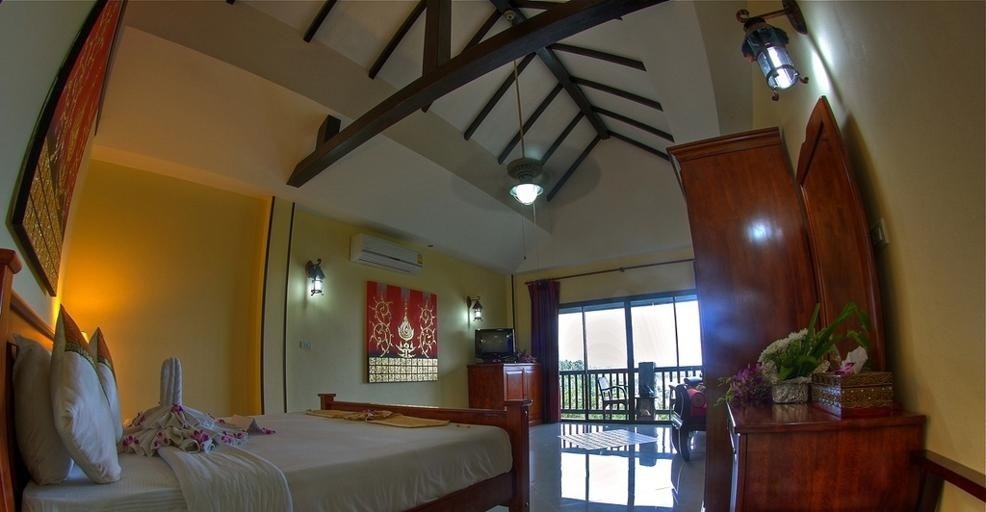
[{"xmin": 634, "ymin": 393, "xmax": 657, "ymax": 421}]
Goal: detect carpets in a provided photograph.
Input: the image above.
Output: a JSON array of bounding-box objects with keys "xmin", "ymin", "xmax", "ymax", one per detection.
[{"xmin": 554, "ymin": 427, "xmax": 659, "ymax": 451}]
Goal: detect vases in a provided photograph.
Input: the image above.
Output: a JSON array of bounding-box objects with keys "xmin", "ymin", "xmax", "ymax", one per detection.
[{"xmin": 769, "ymin": 377, "xmax": 810, "ymax": 403}]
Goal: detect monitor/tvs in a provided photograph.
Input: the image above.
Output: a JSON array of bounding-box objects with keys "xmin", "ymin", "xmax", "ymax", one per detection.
[{"xmin": 475, "ymin": 328, "xmax": 517, "ymax": 363}]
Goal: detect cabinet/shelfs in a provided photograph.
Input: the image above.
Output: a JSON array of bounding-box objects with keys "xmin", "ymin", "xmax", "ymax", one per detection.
[
  {"xmin": 720, "ymin": 398, "xmax": 929, "ymax": 512},
  {"xmin": 465, "ymin": 360, "xmax": 543, "ymax": 428}
]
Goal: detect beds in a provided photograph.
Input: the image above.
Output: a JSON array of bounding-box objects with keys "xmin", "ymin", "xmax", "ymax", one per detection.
[{"xmin": 0, "ymin": 246, "xmax": 534, "ymax": 512}]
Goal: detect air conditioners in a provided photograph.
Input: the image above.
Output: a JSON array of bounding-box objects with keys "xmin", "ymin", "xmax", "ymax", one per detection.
[{"xmin": 347, "ymin": 231, "xmax": 426, "ymax": 278}]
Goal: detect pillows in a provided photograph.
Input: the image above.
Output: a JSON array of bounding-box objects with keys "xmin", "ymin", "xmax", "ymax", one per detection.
[{"xmin": 8, "ymin": 303, "xmax": 132, "ymax": 490}]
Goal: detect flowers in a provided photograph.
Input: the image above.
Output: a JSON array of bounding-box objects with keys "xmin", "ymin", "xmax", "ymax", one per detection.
[{"xmin": 712, "ymin": 298, "xmax": 875, "ymax": 400}]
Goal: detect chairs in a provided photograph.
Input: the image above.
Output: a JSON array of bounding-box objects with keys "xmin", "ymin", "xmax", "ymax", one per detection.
[
  {"xmin": 596, "ymin": 375, "xmax": 632, "ymax": 422},
  {"xmin": 669, "ymin": 378, "xmax": 705, "ymax": 463}
]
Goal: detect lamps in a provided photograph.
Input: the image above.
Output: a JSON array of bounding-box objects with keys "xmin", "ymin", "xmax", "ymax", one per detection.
[
  {"xmin": 731, "ymin": 3, "xmax": 813, "ymax": 103},
  {"xmin": 304, "ymin": 257, "xmax": 328, "ymax": 301},
  {"xmin": 499, "ymin": 8, "xmax": 547, "ymax": 213},
  {"xmin": 465, "ymin": 294, "xmax": 484, "ymax": 325}
]
